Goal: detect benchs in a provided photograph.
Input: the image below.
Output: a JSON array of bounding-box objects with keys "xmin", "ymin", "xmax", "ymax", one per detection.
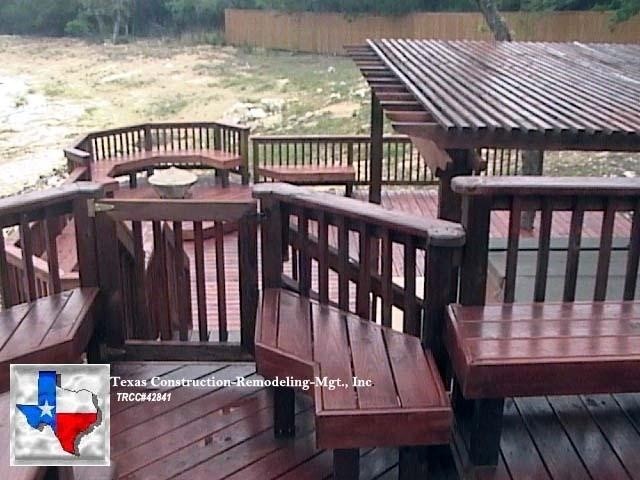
[
  {"xmin": 94, "ymin": 150, "xmax": 240, "ymax": 199},
  {"xmin": 251, "ymin": 183, "xmax": 464, "ymax": 476},
  {"xmin": 258, "ymin": 165, "xmax": 356, "ymax": 198},
  {"xmin": 0, "ymin": 182, "xmax": 104, "ymax": 394},
  {"xmin": 447, "ymin": 176, "xmax": 640, "ymax": 465}
]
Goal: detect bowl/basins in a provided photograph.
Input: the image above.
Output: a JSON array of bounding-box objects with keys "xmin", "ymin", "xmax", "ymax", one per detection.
[{"xmin": 149, "ymin": 172, "xmax": 198, "ymax": 198}]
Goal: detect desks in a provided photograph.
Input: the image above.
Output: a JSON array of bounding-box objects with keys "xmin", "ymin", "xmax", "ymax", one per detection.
[{"xmin": 149, "ymin": 168, "xmax": 198, "ymax": 199}]
